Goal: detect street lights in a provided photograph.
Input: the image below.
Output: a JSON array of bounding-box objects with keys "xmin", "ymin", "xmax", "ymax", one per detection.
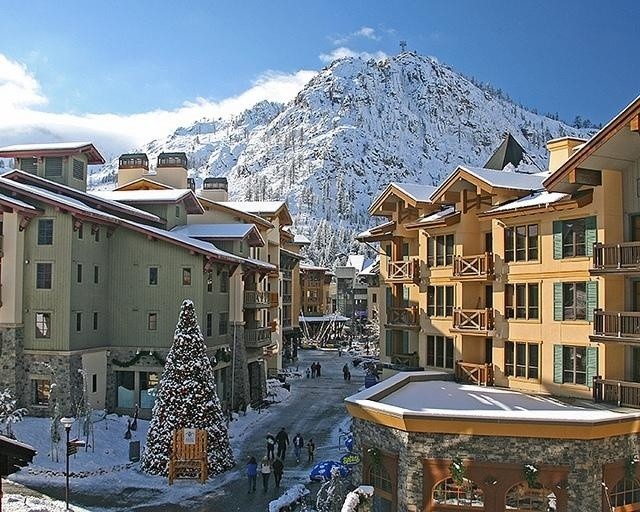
[{"xmin": 60, "ymin": 415, "xmax": 75, "ymax": 508}]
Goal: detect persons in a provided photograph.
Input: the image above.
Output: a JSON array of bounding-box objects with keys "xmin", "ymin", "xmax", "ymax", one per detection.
[
  {"xmin": 305, "ymin": 439, "xmax": 315, "ymax": 464},
  {"xmin": 272, "ymin": 455, "xmax": 284, "ymax": 488},
  {"xmin": 260, "ymin": 456, "xmax": 271, "ymax": 493},
  {"xmin": 265, "ymin": 431, "xmax": 275, "ymax": 462},
  {"xmin": 246, "ymin": 457, "xmax": 258, "ymax": 494},
  {"xmin": 275, "ymin": 427, "xmax": 290, "ymax": 461},
  {"xmin": 347, "ymin": 372, "xmax": 351, "ymax": 381},
  {"xmin": 305, "ymin": 361, "xmax": 322, "ymax": 379},
  {"xmin": 343, "ymin": 363, "xmax": 349, "ymax": 380},
  {"xmin": 292, "ymin": 432, "xmax": 304, "ymax": 463},
  {"xmin": 338, "ymin": 346, "xmax": 342, "ymax": 356}
]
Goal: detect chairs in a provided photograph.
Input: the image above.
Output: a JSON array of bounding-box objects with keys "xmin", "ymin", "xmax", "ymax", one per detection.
[{"xmin": 166, "ymin": 428, "xmax": 208, "ymax": 484}]
